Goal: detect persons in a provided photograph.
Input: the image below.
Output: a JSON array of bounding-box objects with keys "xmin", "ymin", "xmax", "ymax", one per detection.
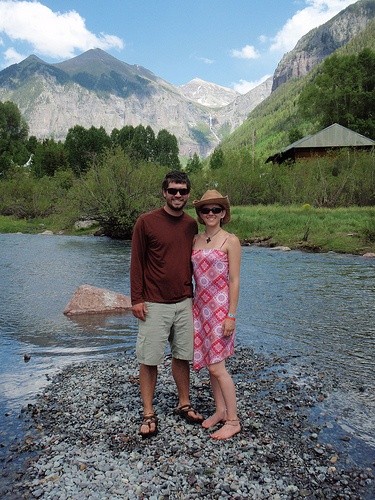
[
  {"xmin": 131, "ymin": 170, "xmax": 206, "ymax": 438},
  {"xmin": 190, "ymin": 190, "xmax": 243, "ymax": 440}
]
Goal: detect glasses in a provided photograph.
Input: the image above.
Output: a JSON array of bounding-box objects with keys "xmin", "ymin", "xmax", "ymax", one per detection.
[
  {"xmin": 200, "ymin": 207, "xmax": 224, "ymax": 214},
  {"xmin": 166, "ymin": 188, "xmax": 191, "ymax": 195}
]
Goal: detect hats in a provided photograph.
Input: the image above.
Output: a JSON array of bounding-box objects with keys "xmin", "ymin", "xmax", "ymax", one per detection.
[{"xmin": 193, "ymin": 189, "xmax": 231, "ymax": 224}]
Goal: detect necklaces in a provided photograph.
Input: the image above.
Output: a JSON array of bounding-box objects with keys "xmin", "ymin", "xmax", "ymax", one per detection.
[{"xmin": 206, "ymin": 228, "xmax": 221, "ymax": 243}]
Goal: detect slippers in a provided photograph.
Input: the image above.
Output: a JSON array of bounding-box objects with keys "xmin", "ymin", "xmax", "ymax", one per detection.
[
  {"xmin": 141, "ymin": 414, "xmax": 158, "ymax": 437},
  {"xmin": 176, "ymin": 404, "xmax": 202, "ymax": 423}
]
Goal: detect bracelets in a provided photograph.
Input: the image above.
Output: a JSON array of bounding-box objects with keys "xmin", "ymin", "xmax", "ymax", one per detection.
[{"xmin": 227, "ymin": 314, "xmax": 236, "ymax": 320}]
